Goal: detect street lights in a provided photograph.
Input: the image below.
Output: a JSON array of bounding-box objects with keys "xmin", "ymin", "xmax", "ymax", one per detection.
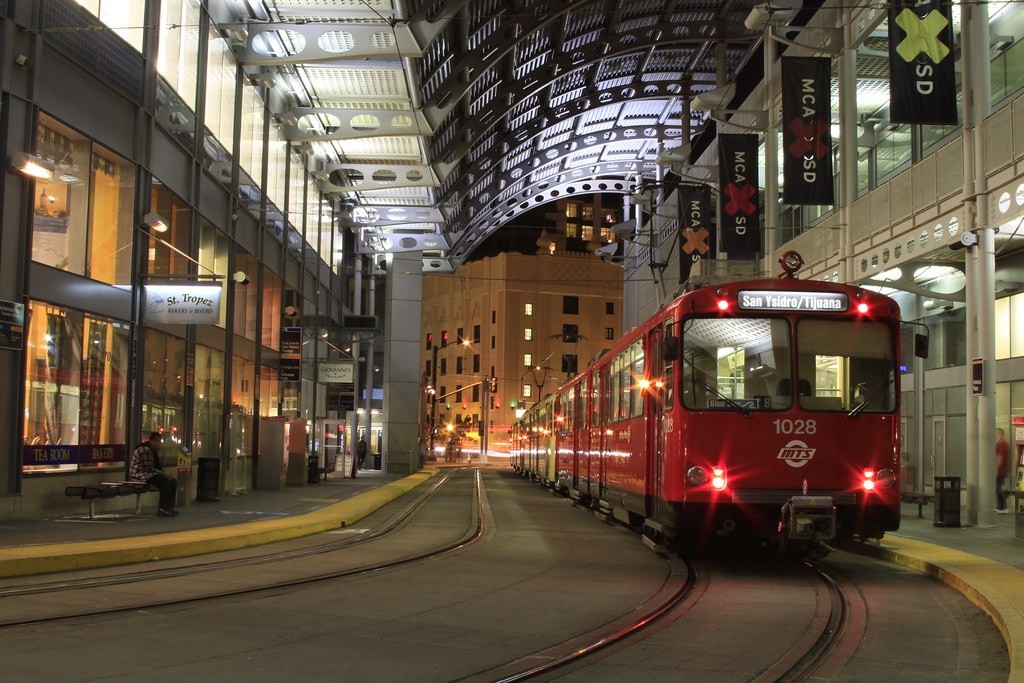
[{"xmin": 430, "ymin": 339, "xmax": 469, "ymax": 454}]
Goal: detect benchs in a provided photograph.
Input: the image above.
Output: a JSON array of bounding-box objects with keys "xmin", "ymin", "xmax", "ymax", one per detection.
[{"xmin": 64, "ymin": 478, "xmax": 159, "ymax": 520}]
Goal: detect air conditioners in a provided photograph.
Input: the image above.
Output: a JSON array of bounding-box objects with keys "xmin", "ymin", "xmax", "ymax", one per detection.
[{"xmin": 342, "ymin": 314, "xmax": 378, "ymax": 331}]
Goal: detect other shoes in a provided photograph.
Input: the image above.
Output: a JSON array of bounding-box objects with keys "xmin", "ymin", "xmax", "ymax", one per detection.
[
  {"xmin": 996, "ymin": 509, "xmax": 1009, "ymax": 514},
  {"xmin": 158, "ymin": 509, "xmax": 179, "ymax": 517}
]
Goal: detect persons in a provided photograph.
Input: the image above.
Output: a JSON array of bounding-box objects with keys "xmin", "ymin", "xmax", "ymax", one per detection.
[
  {"xmin": 357, "ymin": 436, "xmax": 367, "ymax": 471},
  {"xmin": 995, "ymin": 428, "xmax": 1012, "ymax": 515},
  {"xmin": 129, "ymin": 432, "xmax": 179, "ymax": 517},
  {"xmin": 419, "ymin": 439, "xmax": 427, "ymax": 469}
]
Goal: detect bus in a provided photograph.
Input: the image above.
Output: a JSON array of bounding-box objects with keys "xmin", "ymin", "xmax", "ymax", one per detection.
[{"xmin": 508, "ymin": 250, "xmax": 929, "ymax": 560}]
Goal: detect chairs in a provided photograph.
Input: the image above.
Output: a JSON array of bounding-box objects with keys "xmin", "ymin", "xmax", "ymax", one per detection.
[{"xmin": 776, "ymin": 377, "xmax": 813, "ymax": 399}]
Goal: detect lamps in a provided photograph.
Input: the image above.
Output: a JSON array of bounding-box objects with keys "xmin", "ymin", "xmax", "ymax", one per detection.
[
  {"xmin": 744, "ymin": 2, "xmax": 843, "ymax": 53},
  {"xmin": 595, "ymin": 243, "xmax": 635, "ymax": 271},
  {"xmin": 9, "ymin": 153, "xmax": 55, "ymax": 180},
  {"xmin": 690, "ymin": 85, "xmax": 768, "ymax": 131},
  {"xmin": 286, "ymin": 306, "xmax": 298, "ymax": 318},
  {"xmin": 234, "ymin": 272, "xmax": 252, "ymax": 284},
  {"xmin": 654, "ymin": 144, "xmax": 719, "ymax": 184},
  {"xmin": 144, "ymin": 214, "xmax": 170, "ymax": 233},
  {"xmin": 627, "ymin": 191, "xmax": 674, "ymax": 219},
  {"xmin": 609, "ymin": 220, "xmax": 652, "ymax": 250}
]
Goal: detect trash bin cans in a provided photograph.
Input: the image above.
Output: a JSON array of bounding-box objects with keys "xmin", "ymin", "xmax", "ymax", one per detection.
[
  {"xmin": 933, "ymin": 476, "xmax": 962, "ymax": 527},
  {"xmin": 196, "ymin": 456, "xmax": 220, "ymax": 502},
  {"xmin": 374, "ymin": 453, "xmax": 381, "ymax": 470},
  {"xmin": 308, "ymin": 455, "xmax": 319, "ymax": 483}
]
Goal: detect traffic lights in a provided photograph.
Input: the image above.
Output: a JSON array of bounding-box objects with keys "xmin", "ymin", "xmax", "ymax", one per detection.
[
  {"xmin": 491, "ymin": 377, "xmax": 498, "ymax": 392},
  {"xmin": 426, "ymin": 333, "xmax": 432, "ymax": 350},
  {"xmin": 442, "ymin": 330, "xmax": 448, "ymax": 349}
]
